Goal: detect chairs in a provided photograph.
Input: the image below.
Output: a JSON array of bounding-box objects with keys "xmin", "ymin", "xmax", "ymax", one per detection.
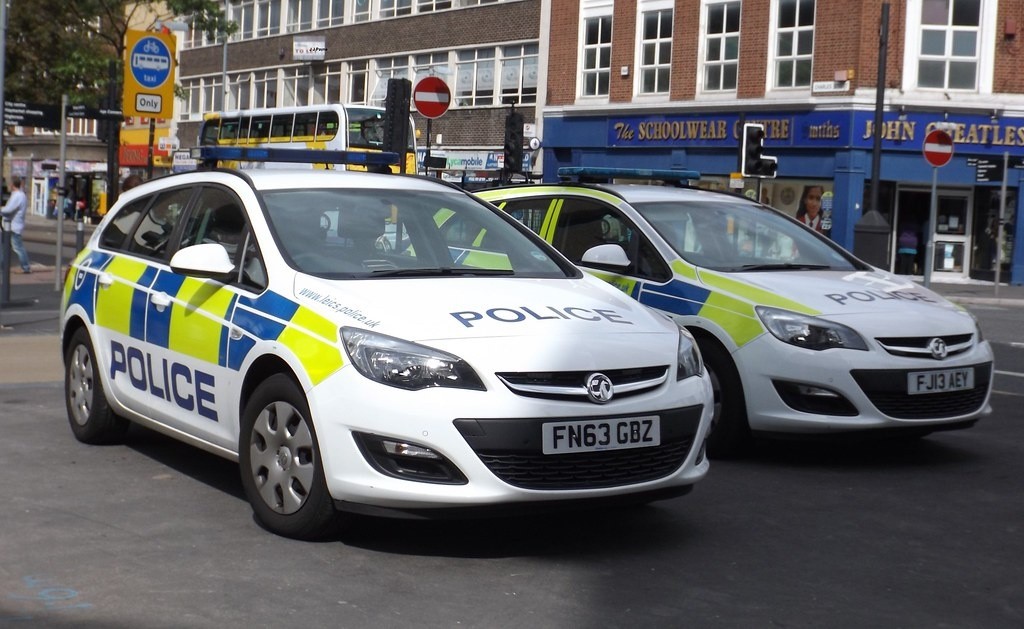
[{"xmin": 337, "ymin": 209, "xmax": 385, "ymax": 266}]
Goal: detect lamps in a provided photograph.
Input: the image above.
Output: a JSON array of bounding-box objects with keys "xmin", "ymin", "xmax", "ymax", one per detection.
[{"xmin": 898, "ymin": 105, "xmax": 996, "ymax": 120}]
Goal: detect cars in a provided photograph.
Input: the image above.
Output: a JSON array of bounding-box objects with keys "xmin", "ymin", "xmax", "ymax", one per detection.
[
  {"xmin": 403, "ymin": 167, "xmax": 996, "ymax": 457},
  {"xmin": 60, "ymin": 146, "xmax": 717, "ymax": 540}
]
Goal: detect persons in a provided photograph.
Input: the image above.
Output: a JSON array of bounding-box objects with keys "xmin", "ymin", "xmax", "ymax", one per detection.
[
  {"xmin": 0, "ymin": 175, "xmax": 31, "ymax": 276},
  {"xmin": 791, "ymin": 185, "xmax": 824, "ymax": 256},
  {"xmin": 53, "ymin": 195, "xmax": 85, "ymax": 221}
]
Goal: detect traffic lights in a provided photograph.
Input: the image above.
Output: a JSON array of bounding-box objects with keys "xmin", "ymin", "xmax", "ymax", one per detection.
[
  {"xmin": 742, "ymin": 124, "xmax": 778, "ymax": 179},
  {"xmin": 503, "ymin": 114, "xmax": 525, "ymax": 170}
]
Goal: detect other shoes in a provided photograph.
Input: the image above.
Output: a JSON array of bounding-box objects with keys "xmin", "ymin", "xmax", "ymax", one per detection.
[{"xmin": 12, "ymin": 267, "xmax": 32, "ymax": 275}]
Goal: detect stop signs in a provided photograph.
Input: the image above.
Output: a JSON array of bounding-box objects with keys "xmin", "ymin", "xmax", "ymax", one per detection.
[
  {"xmin": 414, "ymin": 77, "xmax": 450, "ymax": 118},
  {"xmin": 922, "ymin": 130, "xmax": 955, "ymax": 166}
]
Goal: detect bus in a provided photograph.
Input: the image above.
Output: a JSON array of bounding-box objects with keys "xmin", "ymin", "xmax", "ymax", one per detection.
[{"xmin": 195, "ymin": 102, "xmax": 422, "ymax": 236}]
